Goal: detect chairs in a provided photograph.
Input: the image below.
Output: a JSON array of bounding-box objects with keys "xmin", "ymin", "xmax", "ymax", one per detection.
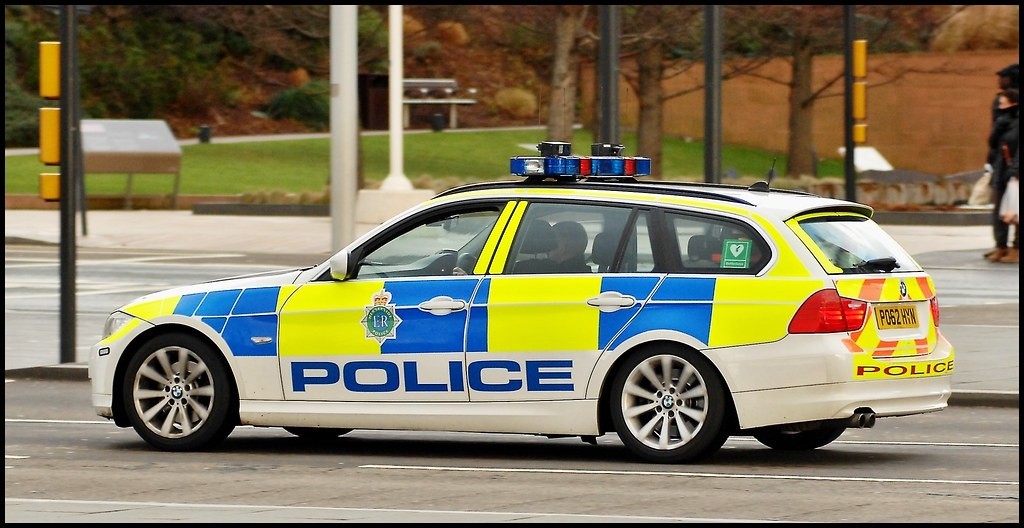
[
  {"xmin": 513, "ymin": 219, "xmax": 559, "ymax": 274},
  {"xmin": 592, "ymin": 233, "xmax": 614, "ymax": 272}
]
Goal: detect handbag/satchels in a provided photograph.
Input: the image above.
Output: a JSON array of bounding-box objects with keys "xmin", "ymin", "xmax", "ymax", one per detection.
[
  {"xmin": 998, "ymin": 177, "xmax": 1019, "ymax": 224},
  {"xmin": 967, "ymin": 172, "xmax": 997, "ymax": 205}
]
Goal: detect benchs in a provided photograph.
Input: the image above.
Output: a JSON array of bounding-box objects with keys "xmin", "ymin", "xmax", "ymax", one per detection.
[{"xmin": 403, "ymin": 78, "xmax": 477, "ymax": 128}]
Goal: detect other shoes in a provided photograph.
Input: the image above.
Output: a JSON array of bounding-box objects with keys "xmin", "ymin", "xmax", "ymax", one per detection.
[
  {"xmin": 990, "ymin": 248, "xmax": 1008, "ymax": 261},
  {"xmin": 1000, "ymin": 247, "xmax": 1019, "ymax": 262}
]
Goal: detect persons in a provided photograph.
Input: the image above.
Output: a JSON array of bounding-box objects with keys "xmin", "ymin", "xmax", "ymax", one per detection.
[
  {"xmin": 983, "ymin": 64, "xmax": 1021, "ymax": 264},
  {"xmin": 452, "ymin": 220, "xmax": 592, "ymax": 276}
]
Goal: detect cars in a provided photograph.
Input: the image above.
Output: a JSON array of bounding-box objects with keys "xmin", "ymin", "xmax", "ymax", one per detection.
[{"xmin": 87, "ymin": 142, "xmax": 954, "ymax": 461}]
